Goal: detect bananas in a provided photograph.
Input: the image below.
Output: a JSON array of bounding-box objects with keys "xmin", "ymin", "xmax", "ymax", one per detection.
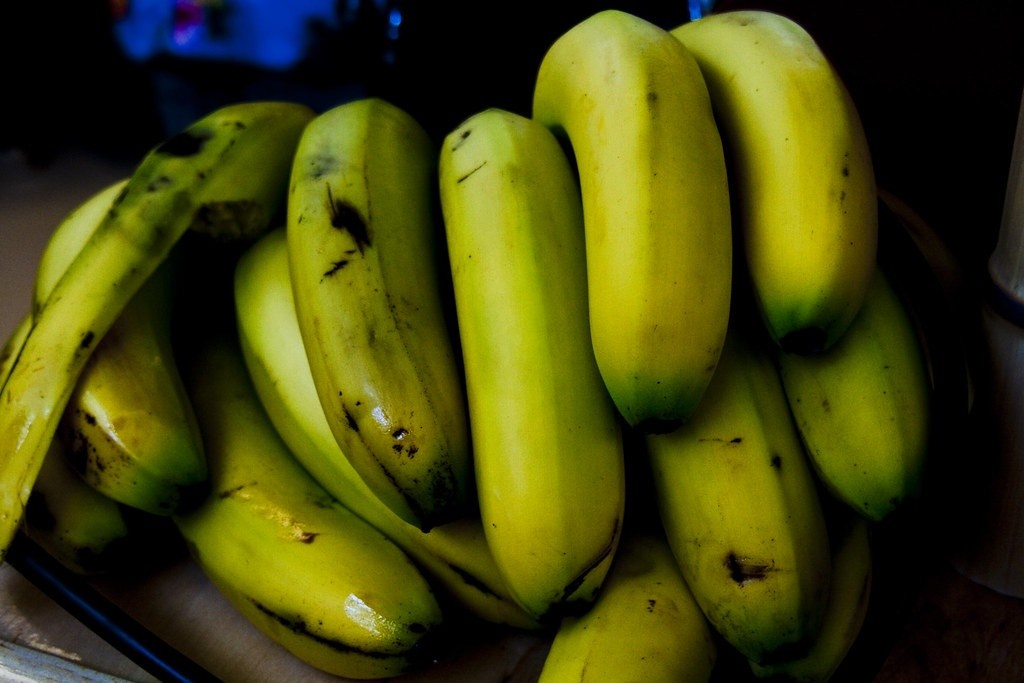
[{"xmin": 0, "ymin": 9, "xmax": 922, "ymax": 683}]
